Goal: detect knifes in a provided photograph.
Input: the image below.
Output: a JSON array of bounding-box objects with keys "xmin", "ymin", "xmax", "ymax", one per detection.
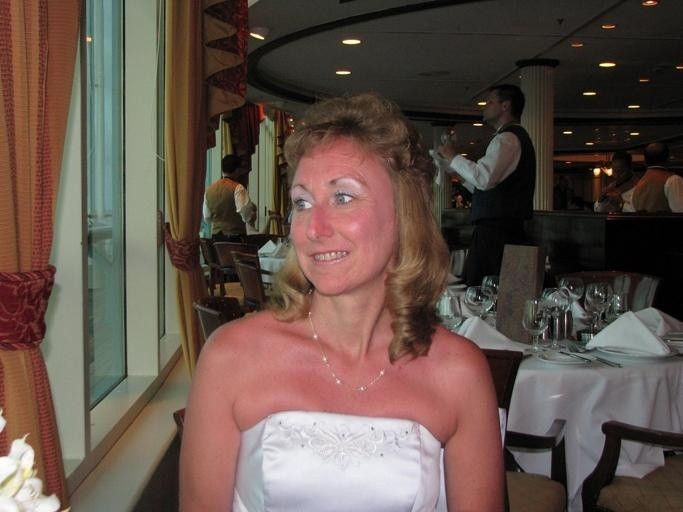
[{"xmin": 557, "ymin": 351, "xmax": 592, "ymax": 363}]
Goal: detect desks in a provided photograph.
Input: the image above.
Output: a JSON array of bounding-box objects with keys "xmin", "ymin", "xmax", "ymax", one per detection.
[
  {"xmin": 258, "ymin": 252, "xmax": 286, "ymax": 285},
  {"xmin": 448, "ymin": 312, "xmax": 683, "ymax": 512}
]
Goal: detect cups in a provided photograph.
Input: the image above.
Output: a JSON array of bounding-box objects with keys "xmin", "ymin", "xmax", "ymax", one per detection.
[
  {"xmin": 437, "ymin": 296, "xmax": 462, "ymax": 331},
  {"xmin": 463, "ymin": 286, "xmax": 495, "ymax": 316}
]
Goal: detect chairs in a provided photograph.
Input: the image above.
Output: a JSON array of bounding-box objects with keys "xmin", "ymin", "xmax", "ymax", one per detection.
[
  {"xmin": 505, "ymin": 418, "xmax": 569, "ymax": 512},
  {"xmin": 213, "ymin": 242, "xmax": 258, "ymax": 296},
  {"xmin": 199, "ymin": 238, "xmax": 227, "ymax": 297},
  {"xmin": 582, "ymin": 420, "xmax": 683, "ymax": 512},
  {"xmin": 229, "ymin": 250, "xmax": 272, "ymax": 309}
]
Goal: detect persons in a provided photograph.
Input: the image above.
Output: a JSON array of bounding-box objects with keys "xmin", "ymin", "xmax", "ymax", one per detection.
[
  {"xmin": 438, "ymin": 83, "xmax": 536, "ymax": 285},
  {"xmin": 632, "ymin": 143, "xmax": 683, "ymax": 213},
  {"xmin": 179, "ymin": 93, "xmax": 506, "ymax": 512},
  {"xmin": 593, "ymin": 151, "xmax": 640, "ymax": 213},
  {"xmin": 203, "ymin": 154, "xmax": 257, "ymax": 241}
]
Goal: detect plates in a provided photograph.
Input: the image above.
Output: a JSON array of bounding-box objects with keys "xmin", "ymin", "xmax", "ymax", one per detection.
[
  {"xmin": 594, "ymin": 345, "xmax": 678, "ymax": 362},
  {"xmin": 536, "ymin": 352, "xmax": 597, "ymax": 365}
]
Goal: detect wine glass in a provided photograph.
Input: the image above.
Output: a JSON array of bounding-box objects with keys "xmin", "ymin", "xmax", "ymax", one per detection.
[
  {"xmin": 481, "ymin": 275, "xmax": 499, "ymax": 320},
  {"xmin": 520, "ymin": 276, "xmax": 628, "ymax": 357},
  {"xmin": 434, "ymin": 128, "xmax": 457, "ymax": 162}
]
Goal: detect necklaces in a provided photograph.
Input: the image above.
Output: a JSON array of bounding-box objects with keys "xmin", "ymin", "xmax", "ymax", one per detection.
[{"xmin": 308, "ymin": 289, "xmax": 392, "ymax": 392}]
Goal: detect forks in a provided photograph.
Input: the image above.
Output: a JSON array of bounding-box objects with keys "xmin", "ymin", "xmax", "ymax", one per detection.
[{"xmin": 567, "ymin": 342, "xmax": 623, "ymax": 369}]
